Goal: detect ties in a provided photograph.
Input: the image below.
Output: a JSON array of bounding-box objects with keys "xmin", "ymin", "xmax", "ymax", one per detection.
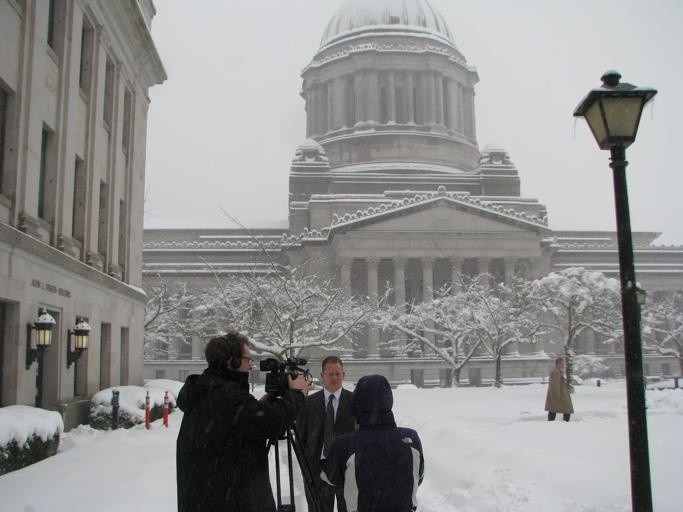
[{"xmin": 322, "ymin": 393, "xmax": 339, "ymax": 462}]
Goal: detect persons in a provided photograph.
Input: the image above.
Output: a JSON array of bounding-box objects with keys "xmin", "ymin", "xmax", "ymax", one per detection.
[
  {"xmin": 319, "ymin": 374, "xmax": 423, "ymax": 512},
  {"xmin": 544, "ymin": 356, "xmax": 573, "ymax": 422},
  {"xmin": 175, "ymin": 330, "xmax": 311, "ymax": 512},
  {"xmin": 294, "ymin": 356, "xmax": 354, "ymax": 511}
]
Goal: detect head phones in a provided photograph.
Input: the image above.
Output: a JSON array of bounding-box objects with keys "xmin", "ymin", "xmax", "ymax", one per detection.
[{"xmin": 220, "ymin": 335, "xmax": 241, "ymax": 369}]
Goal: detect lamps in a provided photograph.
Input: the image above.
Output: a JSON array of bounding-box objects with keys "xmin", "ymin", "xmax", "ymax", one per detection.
[
  {"xmin": 65, "ymin": 319, "xmax": 91, "ymax": 369},
  {"xmin": 25, "ymin": 310, "xmax": 56, "ymax": 370}
]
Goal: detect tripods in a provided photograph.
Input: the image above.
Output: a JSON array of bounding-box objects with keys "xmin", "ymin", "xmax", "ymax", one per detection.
[{"xmin": 264, "ymin": 425, "xmax": 326, "ymax": 512}]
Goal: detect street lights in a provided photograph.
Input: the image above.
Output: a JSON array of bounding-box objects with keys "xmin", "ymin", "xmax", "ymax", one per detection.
[{"xmin": 570, "ymin": 67, "xmax": 660, "ymax": 512}]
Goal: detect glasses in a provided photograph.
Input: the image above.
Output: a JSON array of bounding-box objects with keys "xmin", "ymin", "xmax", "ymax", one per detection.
[{"xmin": 240, "ymin": 356, "xmax": 254, "ymax": 365}]
